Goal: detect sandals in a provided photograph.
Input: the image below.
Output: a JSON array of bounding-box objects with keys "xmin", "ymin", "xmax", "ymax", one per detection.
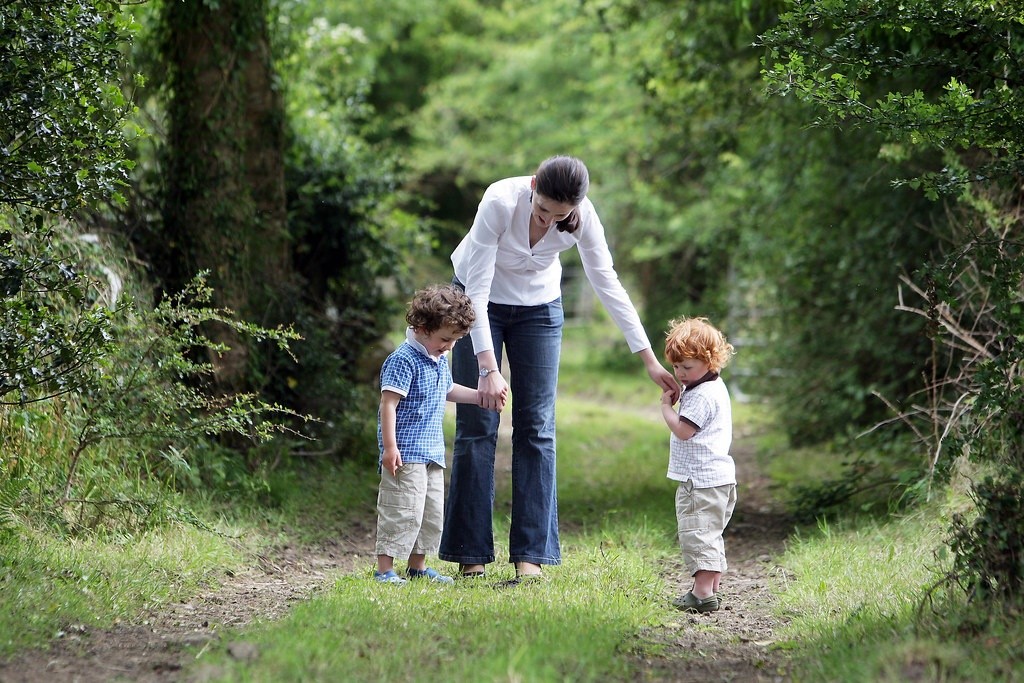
[
  {"xmin": 672, "ymin": 589, "xmax": 718, "ymax": 611},
  {"xmin": 714, "ymin": 592, "xmax": 721, "ymax": 609}
]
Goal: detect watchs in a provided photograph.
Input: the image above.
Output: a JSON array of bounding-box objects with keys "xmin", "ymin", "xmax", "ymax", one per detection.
[{"xmin": 478, "ymin": 367, "xmax": 500, "ymax": 378}]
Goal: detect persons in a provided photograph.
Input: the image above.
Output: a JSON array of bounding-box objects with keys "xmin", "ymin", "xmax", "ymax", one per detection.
[
  {"xmin": 438, "ymin": 154, "xmax": 680, "ymax": 592},
  {"xmin": 659, "ymin": 316, "xmax": 738, "ymax": 614},
  {"xmin": 369, "ymin": 284, "xmax": 507, "ymax": 587}
]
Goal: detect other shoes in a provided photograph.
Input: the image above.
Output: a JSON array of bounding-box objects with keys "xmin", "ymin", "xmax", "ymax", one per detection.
[
  {"xmin": 459, "ymin": 563, "xmax": 485, "ymax": 581},
  {"xmin": 406, "ymin": 567, "xmax": 454, "ymax": 584},
  {"xmin": 374, "ymin": 571, "xmax": 405, "ymax": 585},
  {"xmin": 492, "ymin": 575, "xmax": 542, "ymax": 590}
]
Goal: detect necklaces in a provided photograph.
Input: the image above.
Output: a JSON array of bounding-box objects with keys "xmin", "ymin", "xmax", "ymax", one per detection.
[{"xmin": 535, "ymin": 224, "xmax": 554, "ymax": 244}]
